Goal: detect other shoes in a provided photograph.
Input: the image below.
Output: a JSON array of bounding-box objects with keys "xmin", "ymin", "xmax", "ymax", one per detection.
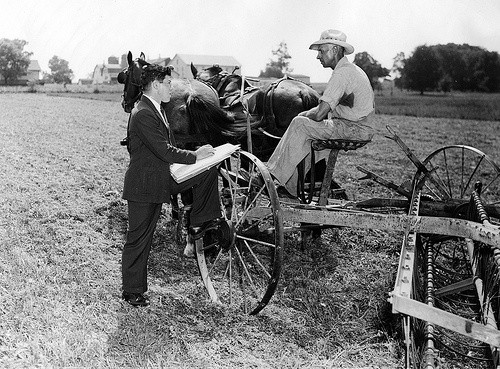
[
  {"xmin": 238, "ymin": 168, "xmax": 268, "ymax": 195},
  {"xmin": 276, "ymin": 185, "xmax": 296, "ymax": 200}
]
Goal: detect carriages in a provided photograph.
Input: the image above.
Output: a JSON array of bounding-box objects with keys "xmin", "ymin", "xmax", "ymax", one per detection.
[{"xmin": 115, "ymin": 51, "xmax": 500, "ymax": 369}]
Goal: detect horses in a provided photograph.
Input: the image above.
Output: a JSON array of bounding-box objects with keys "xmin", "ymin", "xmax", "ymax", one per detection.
[
  {"xmin": 187, "ymin": 59, "xmax": 332, "ymax": 237},
  {"xmin": 117, "ymin": 50, "xmax": 226, "ymax": 261}
]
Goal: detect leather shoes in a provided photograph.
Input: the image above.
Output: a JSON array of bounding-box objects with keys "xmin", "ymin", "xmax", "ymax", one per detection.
[
  {"xmin": 122, "ymin": 289, "xmax": 149, "ymax": 306},
  {"xmin": 189, "ymin": 220, "xmax": 212, "ymax": 240}
]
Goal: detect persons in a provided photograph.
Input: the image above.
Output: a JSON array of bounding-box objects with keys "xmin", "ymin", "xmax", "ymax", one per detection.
[
  {"xmin": 122, "ymin": 64, "xmax": 224, "ymax": 306},
  {"xmin": 238, "ymin": 29, "xmax": 374, "ymax": 198}
]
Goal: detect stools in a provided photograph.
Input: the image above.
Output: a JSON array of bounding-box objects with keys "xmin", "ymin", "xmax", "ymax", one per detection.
[{"xmin": 307, "ymin": 137, "xmax": 372, "ymax": 208}]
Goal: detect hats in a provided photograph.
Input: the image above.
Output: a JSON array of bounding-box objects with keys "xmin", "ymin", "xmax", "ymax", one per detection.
[{"xmin": 309, "ymin": 30, "xmax": 355, "ymax": 55}]
